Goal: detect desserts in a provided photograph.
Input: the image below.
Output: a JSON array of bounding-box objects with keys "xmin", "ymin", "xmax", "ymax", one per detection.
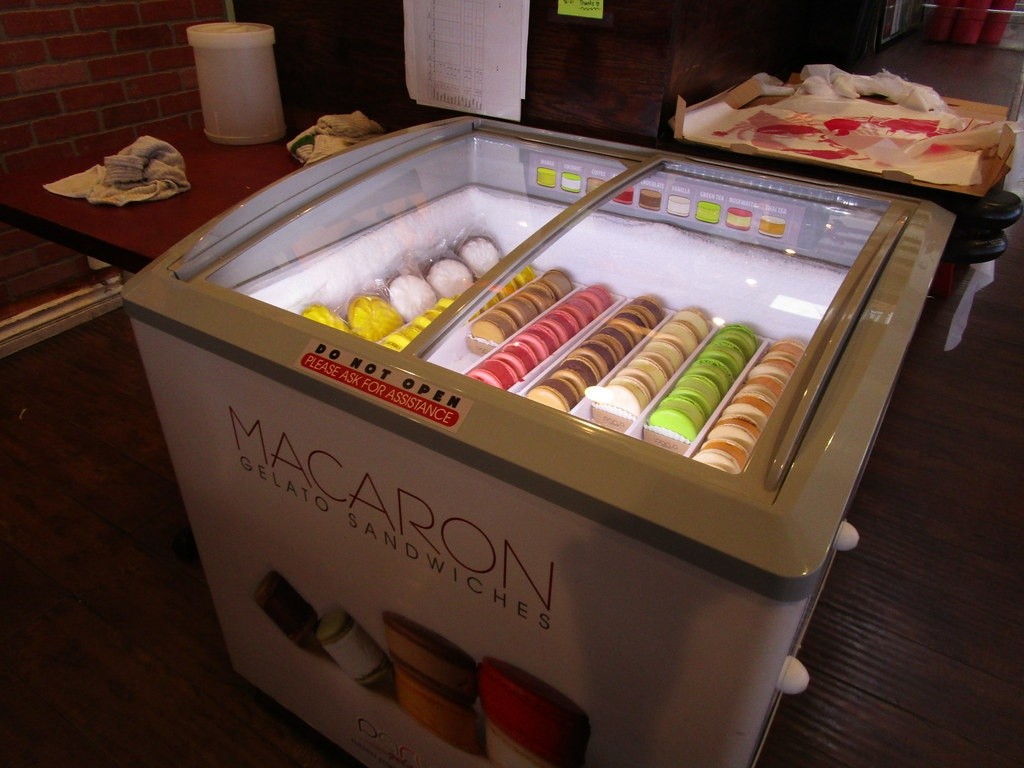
[{"xmin": 297, "ymin": 236, "xmax": 806, "ymax": 476}]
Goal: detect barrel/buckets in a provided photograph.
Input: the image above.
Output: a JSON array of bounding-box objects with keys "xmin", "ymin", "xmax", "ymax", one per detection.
[{"xmin": 186, "ymin": 19, "xmax": 287, "ymax": 144}]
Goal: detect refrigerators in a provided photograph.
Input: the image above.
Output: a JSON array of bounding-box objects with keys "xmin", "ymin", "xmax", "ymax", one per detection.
[{"xmin": 120, "ymin": 115, "xmax": 955, "ymax": 768}]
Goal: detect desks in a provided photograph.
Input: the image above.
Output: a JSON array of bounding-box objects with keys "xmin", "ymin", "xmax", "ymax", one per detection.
[
  {"xmin": 677, "ymin": 45, "xmax": 1024, "ymax": 201},
  {"xmin": 1, "ymin": 108, "xmax": 390, "ymax": 274}
]
